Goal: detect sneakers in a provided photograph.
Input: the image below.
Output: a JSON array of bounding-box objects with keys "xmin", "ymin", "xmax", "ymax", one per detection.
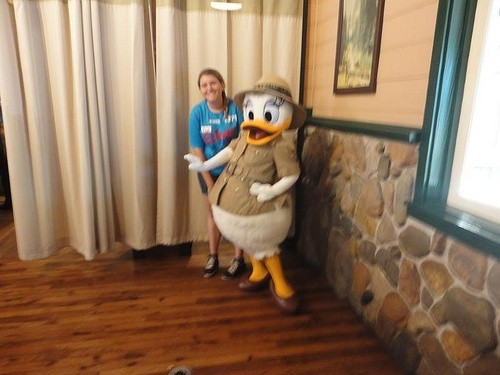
[
  {"xmin": 220, "ymin": 257, "xmax": 244, "ymax": 281},
  {"xmin": 203, "ymin": 254, "xmax": 218, "ymax": 278}
]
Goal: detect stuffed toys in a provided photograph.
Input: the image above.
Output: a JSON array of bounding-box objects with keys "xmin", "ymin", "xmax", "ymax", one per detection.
[{"xmin": 183, "ymin": 73, "xmax": 306, "ymax": 316}]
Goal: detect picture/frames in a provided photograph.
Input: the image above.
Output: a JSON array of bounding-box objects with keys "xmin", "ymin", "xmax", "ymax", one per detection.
[{"xmin": 333, "ymin": 0, "xmax": 385, "ymax": 97}]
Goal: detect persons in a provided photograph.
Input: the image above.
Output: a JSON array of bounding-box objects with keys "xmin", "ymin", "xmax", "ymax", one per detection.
[{"xmin": 188, "ymin": 69, "xmax": 246, "ymax": 279}]
